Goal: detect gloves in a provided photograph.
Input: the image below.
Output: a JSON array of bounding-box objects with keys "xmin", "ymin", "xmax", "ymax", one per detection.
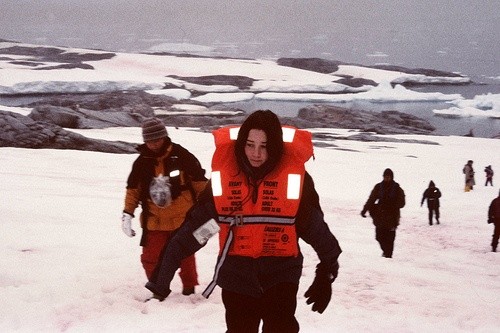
[
  {"xmin": 361, "ymin": 209, "xmax": 367, "ymax": 218},
  {"xmin": 332, "ymin": 261, "xmax": 339, "ymax": 283},
  {"xmin": 303, "ymin": 262, "xmax": 331, "ymax": 314},
  {"xmin": 122, "ymin": 210, "xmax": 136, "ymax": 238}
]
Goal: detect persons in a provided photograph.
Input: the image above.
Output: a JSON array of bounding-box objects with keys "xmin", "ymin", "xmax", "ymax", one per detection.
[
  {"xmin": 421, "ymin": 180, "xmax": 442, "ymax": 226},
  {"xmin": 144, "ymin": 110, "xmax": 342, "ymax": 333},
  {"xmin": 361, "ymin": 168, "xmax": 405, "ymax": 258},
  {"xmin": 122, "ymin": 117, "xmax": 210, "ymax": 303},
  {"xmin": 463, "ymin": 160, "xmax": 476, "ymax": 192},
  {"xmin": 484, "ymin": 165, "xmax": 494, "ymax": 186},
  {"xmin": 488, "ymin": 189, "xmax": 500, "ymax": 252}
]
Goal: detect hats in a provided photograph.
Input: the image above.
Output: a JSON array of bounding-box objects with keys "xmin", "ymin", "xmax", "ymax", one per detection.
[
  {"xmin": 429, "ymin": 180, "xmax": 435, "ymax": 186},
  {"xmin": 142, "ymin": 118, "xmax": 168, "ymax": 141},
  {"xmin": 384, "ymin": 169, "xmax": 393, "ymax": 177}
]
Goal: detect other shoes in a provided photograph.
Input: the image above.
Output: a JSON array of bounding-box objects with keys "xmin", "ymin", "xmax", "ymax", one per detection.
[{"xmin": 183, "ymin": 286, "xmax": 195, "ymax": 295}]
